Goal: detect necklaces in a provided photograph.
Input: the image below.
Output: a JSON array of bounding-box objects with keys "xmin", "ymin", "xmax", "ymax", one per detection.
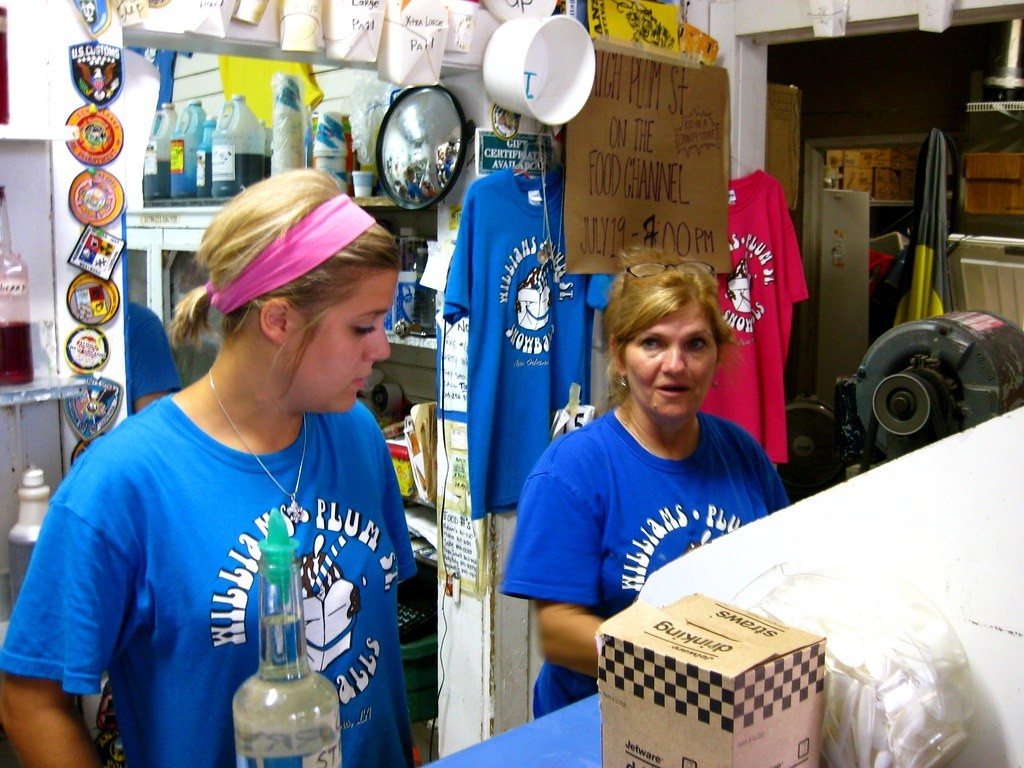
[
  {"xmin": 209, "ymin": 368, "xmax": 307, "ymax": 525},
  {"xmin": 620, "ymin": 417, "xmax": 658, "ymax": 457}
]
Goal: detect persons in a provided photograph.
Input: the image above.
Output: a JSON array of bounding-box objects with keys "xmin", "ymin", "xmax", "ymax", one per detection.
[
  {"xmin": 0, "ymin": 169, "xmax": 422, "ymax": 768},
  {"xmin": 128, "ymin": 301, "xmax": 181, "ymax": 417},
  {"xmin": 498, "ymin": 251, "xmax": 791, "ymax": 720}
]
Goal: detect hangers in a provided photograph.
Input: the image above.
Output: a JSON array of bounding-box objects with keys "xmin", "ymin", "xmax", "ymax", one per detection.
[
  {"xmin": 514, "ymin": 124, "xmax": 562, "ymax": 177},
  {"xmin": 730, "ymin": 155, "xmax": 757, "ymax": 175}
]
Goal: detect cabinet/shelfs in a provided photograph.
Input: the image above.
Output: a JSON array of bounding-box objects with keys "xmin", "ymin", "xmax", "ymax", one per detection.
[
  {"xmin": 348, "ymin": 195, "xmax": 438, "ymax": 566},
  {"xmin": 125, "ymin": 204, "xmax": 225, "ymax": 327},
  {"xmin": 0, "ymin": 122, "xmax": 91, "ymax": 409}
]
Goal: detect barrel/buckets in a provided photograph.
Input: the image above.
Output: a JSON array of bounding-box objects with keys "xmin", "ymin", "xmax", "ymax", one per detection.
[{"xmin": 137, "ymin": 94, "xmax": 271, "ymax": 201}]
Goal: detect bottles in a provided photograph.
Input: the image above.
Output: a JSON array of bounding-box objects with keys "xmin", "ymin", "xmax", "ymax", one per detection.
[
  {"xmin": 229, "ymin": 503, "xmax": 345, "ymax": 768},
  {"xmin": 409, "ymin": 248, "xmax": 436, "ymax": 330},
  {"xmin": 0, "ymin": 239, "xmax": 35, "ymax": 386},
  {"xmin": 197, "ymin": 118, "xmax": 215, "ymax": 194},
  {"xmin": 8, "ymin": 468, "xmax": 51, "ymax": 613}
]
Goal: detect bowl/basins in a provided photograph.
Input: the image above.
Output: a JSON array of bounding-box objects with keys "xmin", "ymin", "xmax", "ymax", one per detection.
[{"xmin": 482, "ymin": 14, "xmax": 596, "ymax": 127}]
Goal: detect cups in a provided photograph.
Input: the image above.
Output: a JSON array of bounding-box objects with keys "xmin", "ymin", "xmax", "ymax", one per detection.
[
  {"xmin": 271, "ymin": 73, "xmax": 307, "ymax": 176},
  {"xmin": 312, "ymin": 110, "xmax": 348, "ymax": 196},
  {"xmin": 352, "ymin": 170, "xmax": 374, "ymax": 198}
]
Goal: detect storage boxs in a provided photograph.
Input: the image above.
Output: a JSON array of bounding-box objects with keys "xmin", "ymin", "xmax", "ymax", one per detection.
[
  {"xmin": 963, "ymin": 151, "xmax": 1024, "ymax": 181},
  {"xmin": 826, "ymin": 147, "xmax": 921, "ymax": 201},
  {"xmin": 964, "ymin": 178, "xmax": 1024, "ymax": 216},
  {"xmin": 596, "ymin": 591, "xmax": 827, "ymax": 768}
]
